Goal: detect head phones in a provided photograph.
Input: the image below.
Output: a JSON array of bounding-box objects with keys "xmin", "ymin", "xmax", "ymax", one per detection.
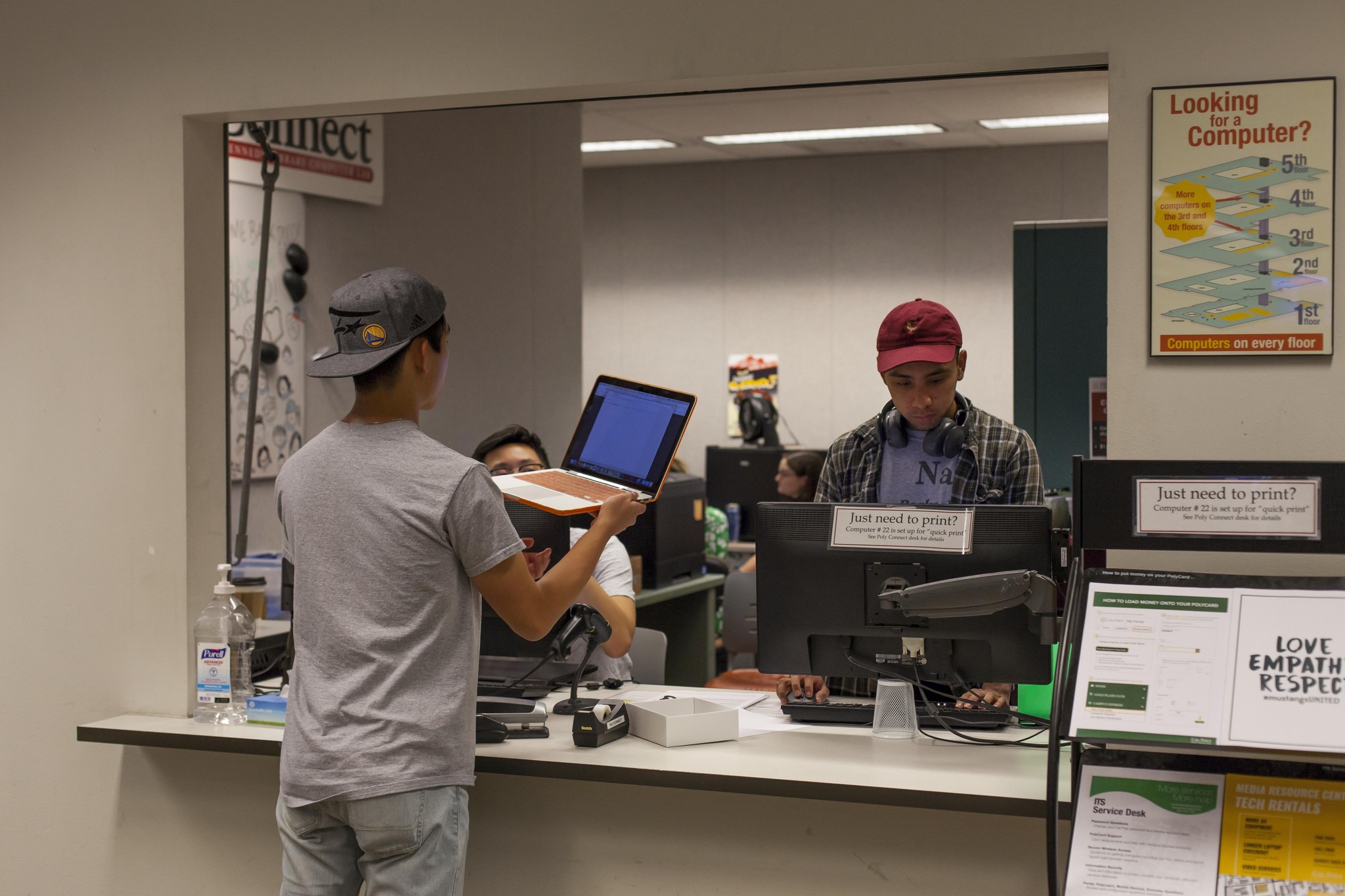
[{"xmin": 876, "ymin": 391, "xmax": 971, "ymax": 458}]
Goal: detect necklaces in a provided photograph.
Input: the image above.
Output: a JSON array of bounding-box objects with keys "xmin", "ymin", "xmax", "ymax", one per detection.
[{"xmin": 341, "ymin": 418, "xmax": 407, "ymax": 425}]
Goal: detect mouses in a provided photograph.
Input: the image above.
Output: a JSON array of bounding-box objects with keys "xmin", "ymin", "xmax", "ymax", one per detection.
[{"xmin": 474, "ymin": 714, "xmax": 511, "ymax": 744}]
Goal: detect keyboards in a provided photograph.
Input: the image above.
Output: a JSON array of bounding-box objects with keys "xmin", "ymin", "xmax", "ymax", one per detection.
[{"xmin": 780, "ymin": 698, "xmax": 1015, "ymax": 731}]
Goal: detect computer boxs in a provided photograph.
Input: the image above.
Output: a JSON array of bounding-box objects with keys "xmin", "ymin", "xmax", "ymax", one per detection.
[
  {"xmin": 1069, "ymin": 453, "xmax": 1343, "ymax": 554},
  {"xmin": 609, "ymin": 471, "xmax": 705, "ymax": 588},
  {"xmin": 704, "ymin": 444, "xmax": 828, "ymax": 536}
]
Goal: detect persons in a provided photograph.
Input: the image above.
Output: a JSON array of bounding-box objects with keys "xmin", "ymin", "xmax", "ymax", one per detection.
[
  {"xmin": 715, "ymin": 452, "xmax": 827, "ymax": 649},
  {"xmin": 777, "ymin": 298, "xmax": 1047, "ymax": 722},
  {"xmin": 275, "ymin": 265, "xmax": 648, "ymax": 896},
  {"xmin": 472, "ymin": 427, "xmax": 637, "ymax": 685}
]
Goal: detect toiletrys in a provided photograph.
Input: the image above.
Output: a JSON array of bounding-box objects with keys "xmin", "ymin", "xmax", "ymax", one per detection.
[{"xmin": 193, "ymin": 562, "xmax": 256, "ymax": 726}]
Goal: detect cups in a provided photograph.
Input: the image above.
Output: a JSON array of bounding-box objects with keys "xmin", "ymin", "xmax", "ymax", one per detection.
[{"xmin": 233, "ymin": 576, "xmax": 266, "ymax": 626}]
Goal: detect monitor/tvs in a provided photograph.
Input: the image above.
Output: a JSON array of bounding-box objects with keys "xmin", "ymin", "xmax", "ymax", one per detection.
[
  {"xmin": 475, "ymin": 500, "xmax": 600, "ymax": 721},
  {"xmin": 753, "ymin": 501, "xmax": 1059, "ymax": 742}
]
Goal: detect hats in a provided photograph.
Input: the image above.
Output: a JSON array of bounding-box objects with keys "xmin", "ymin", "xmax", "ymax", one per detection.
[
  {"xmin": 877, "ymin": 297, "xmax": 963, "ymax": 373},
  {"xmin": 306, "ymin": 267, "xmax": 447, "ymax": 378}
]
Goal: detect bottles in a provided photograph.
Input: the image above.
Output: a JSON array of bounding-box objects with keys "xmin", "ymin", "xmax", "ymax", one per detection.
[{"xmin": 725, "ymin": 503, "xmax": 740, "ymax": 543}]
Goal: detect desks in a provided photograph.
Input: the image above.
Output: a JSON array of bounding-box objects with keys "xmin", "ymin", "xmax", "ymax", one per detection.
[{"xmin": 634, "ymin": 571, "xmax": 726, "ymax": 689}]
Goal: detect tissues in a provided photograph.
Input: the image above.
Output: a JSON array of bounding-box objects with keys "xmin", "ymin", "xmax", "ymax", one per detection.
[{"xmin": 246, "ymin": 669, "xmax": 292, "ymax": 726}]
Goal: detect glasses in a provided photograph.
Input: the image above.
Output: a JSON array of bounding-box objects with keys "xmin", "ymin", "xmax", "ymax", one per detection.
[{"xmin": 489, "ymin": 463, "xmax": 546, "ymax": 477}]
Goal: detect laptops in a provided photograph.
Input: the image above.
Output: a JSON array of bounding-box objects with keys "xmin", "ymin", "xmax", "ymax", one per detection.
[{"xmin": 488, "ymin": 375, "xmax": 698, "ymax": 517}]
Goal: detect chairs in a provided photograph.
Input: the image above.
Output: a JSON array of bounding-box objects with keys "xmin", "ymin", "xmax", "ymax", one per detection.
[{"xmin": 628, "ymin": 627, "xmax": 667, "ymax": 686}]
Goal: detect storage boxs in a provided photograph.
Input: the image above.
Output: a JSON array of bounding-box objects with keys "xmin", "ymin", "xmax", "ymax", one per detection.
[{"xmin": 627, "ymin": 698, "xmax": 739, "ymax": 748}]
